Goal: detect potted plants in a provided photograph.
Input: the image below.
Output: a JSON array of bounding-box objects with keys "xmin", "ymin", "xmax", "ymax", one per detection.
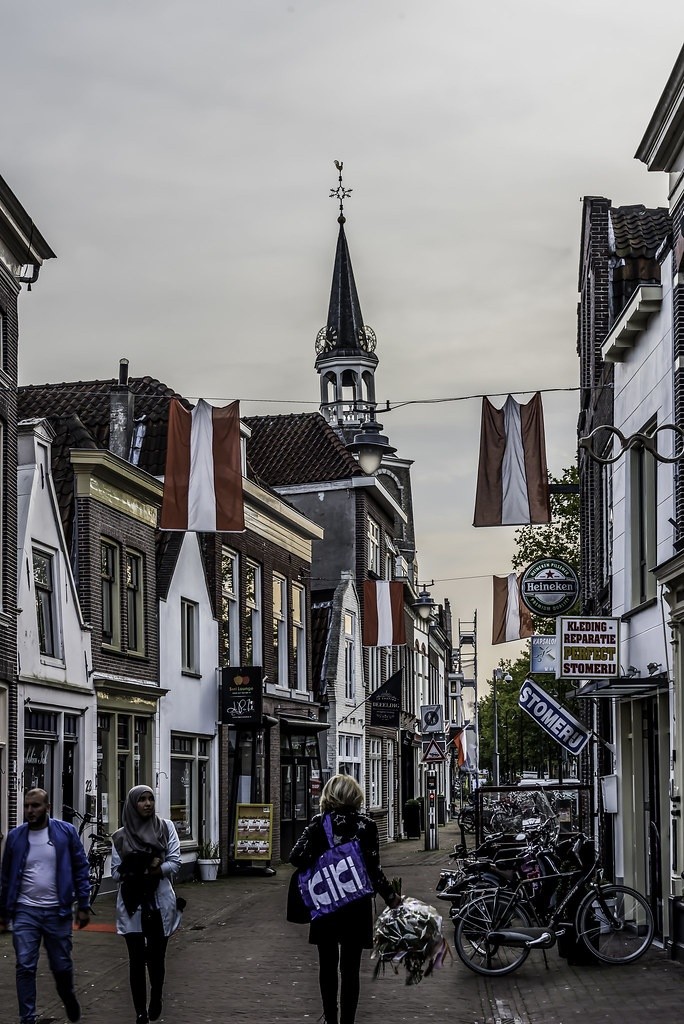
[
  {"xmin": 555, "ymin": 860, "xmax": 597, "ymax": 956},
  {"xmin": 403, "ymin": 799, "xmax": 423, "ymax": 839},
  {"xmin": 586, "ymin": 877, "xmax": 618, "ymax": 934},
  {"xmin": 196, "ymin": 838, "xmax": 221, "ymax": 881}
]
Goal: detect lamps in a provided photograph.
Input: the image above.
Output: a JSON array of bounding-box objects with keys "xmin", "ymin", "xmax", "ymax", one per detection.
[
  {"xmin": 627, "ymin": 665, "xmax": 640, "ymax": 676},
  {"xmin": 410, "ymin": 579, "xmax": 438, "ymax": 619},
  {"xmin": 647, "ymin": 663, "xmax": 662, "ymax": 675},
  {"xmin": 344, "ymin": 400, "xmax": 398, "ymax": 476}
]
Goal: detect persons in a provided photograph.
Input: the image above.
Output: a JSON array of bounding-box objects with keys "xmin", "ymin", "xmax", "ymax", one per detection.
[
  {"xmin": 290, "ymin": 774, "xmax": 402, "ymax": 1024},
  {"xmin": 110, "ymin": 785, "xmax": 182, "ymax": 1024},
  {"xmin": 0, "ymin": 789, "xmax": 91, "ymax": 1024}
]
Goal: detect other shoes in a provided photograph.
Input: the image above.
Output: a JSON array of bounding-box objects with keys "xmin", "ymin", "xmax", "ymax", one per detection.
[
  {"xmin": 148, "ymin": 998, "xmax": 162, "ymax": 1021},
  {"xmin": 65, "ymin": 999, "xmax": 80, "ymax": 1023},
  {"xmin": 136, "ymin": 1013, "xmax": 148, "ymax": 1024}
]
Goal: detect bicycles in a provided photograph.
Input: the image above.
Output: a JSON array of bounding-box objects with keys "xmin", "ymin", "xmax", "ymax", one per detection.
[
  {"xmin": 451, "ymin": 831, "xmax": 656, "ymax": 977},
  {"xmin": 435, "ymin": 771, "xmax": 602, "ymax": 959},
  {"xmin": 59, "ymin": 803, "xmax": 113, "ymax": 920}
]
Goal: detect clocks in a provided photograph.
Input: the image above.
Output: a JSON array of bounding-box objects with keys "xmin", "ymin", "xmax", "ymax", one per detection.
[
  {"xmin": 314, "ymin": 325, "xmax": 337, "ymax": 355},
  {"xmin": 357, "ymin": 326, "xmax": 377, "ymax": 353}
]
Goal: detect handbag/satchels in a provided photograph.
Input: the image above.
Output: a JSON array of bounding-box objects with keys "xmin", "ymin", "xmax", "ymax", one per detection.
[
  {"xmin": 286, "ymin": 870, "xmax": 310, "ymax": 924},
  {"xmin": 297, "ymin": 815, "xmax": 375, "ymax": 921}
]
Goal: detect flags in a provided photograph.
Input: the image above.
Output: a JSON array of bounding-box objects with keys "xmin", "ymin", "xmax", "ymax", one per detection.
[
  {"xmin": 491, "ymin": 574, "xmax": 532, "ymax": 644},
  {"xmin": 453, "ymin": 729, "xmax": 466, "ymax": 766},
  {"xmin": 472, "ymin": 390, "xmax": 551, "ymax": 526},
  {"xmin": 158, "ymin": 398, "xmax": 244, "ymax": 533},
  {"xmin": 362, "ymin": 580, "xmax": 406, "ymax": 647}
]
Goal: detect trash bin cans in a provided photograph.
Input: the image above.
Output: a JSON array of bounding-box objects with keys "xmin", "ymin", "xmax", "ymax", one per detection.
[
  {"xmin": 405, "ymin": 799, "xmax": 422, "ymax": 839},
  {"xmin": 438, "ymin": 795, "xmax": 447, "ymax": 827}
]
